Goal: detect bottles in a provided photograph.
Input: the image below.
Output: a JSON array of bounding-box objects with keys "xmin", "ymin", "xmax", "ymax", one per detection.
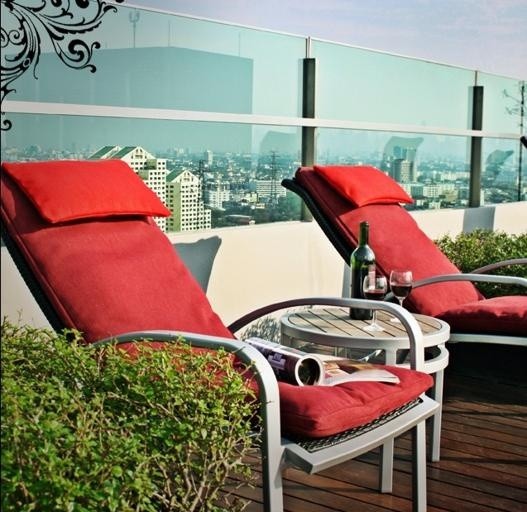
[{"xmin": 347, "ymin": 219, "xmax": 374, "ymax": 321}]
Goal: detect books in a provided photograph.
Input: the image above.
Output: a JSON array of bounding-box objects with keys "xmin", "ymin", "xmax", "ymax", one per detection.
[{"xmin": 243, "ymin": 337, "xmax": 400, "ymax": 388}]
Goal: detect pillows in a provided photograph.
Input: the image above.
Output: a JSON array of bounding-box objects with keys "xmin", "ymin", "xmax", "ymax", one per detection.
[
  {"xmin": 1, "ymin": 161, "xmax": 171, "ymax": 225},
  {"xmin": 313, "ymin": 164, "xmax": 417, "ymax": 207}
]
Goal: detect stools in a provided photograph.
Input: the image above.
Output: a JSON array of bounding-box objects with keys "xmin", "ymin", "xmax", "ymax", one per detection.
[{"xmin": 281, "ymin": 308, "xmax": 450, "ymax": 494}]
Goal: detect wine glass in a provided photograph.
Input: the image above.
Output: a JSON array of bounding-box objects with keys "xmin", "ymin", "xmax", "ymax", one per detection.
[
  {"xmin": 388, "ymin": 270, "xmax": 413, "ymax": 322},
  {"xmin": 362, "ymin": 273, "xmax": 387, "ymax": 332}
]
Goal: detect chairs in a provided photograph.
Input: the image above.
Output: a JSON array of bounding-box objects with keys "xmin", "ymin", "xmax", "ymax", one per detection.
[
  {"xmin": 281, "ymin": 166, "xmax": 527, "ymax": 364},
  {"xmin": 2, "ymin": 160, "xmax": 441, "ymax": 512}
]
